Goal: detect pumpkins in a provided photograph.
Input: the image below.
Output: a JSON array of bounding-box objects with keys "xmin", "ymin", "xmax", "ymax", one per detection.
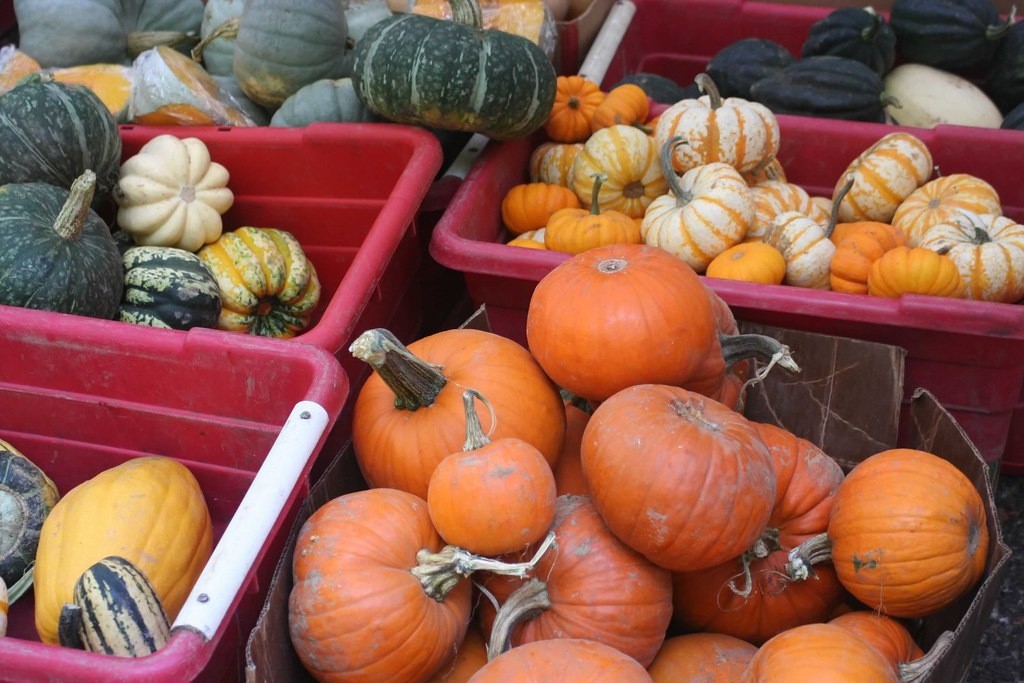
[{"xmin": 0, "ymin": 0, "xmax": 1024, "ymax": 683}]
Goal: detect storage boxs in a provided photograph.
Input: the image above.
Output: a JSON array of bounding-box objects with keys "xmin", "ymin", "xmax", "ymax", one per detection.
[
  {"xmin": 577, "ymin": 0, "xmax": 1024, "ymax": 94},
  {"xmin": 0, "ymin": 304, "xmax": 350, "ymax": 683},
  {"xmin": 430, "ymin": 104, "xmax": 1024, "ymax": 461},
  {"xmin": 118, "ymin": 123, "xmax": 442, "ymax": 391}
]
